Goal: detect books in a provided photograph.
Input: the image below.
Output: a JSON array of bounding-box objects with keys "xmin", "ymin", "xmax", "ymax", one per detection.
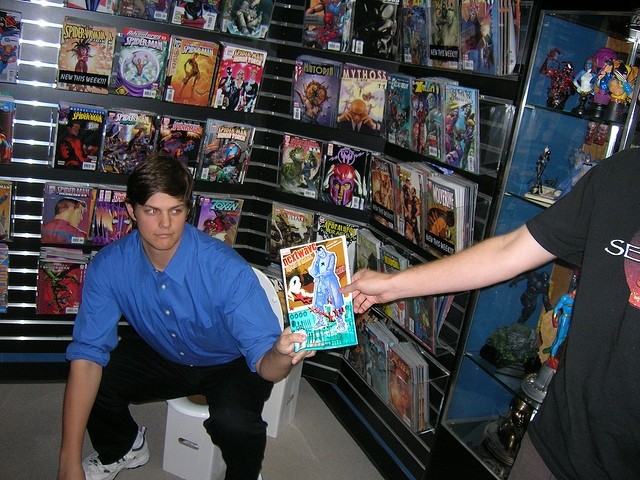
[
  {"xmin": 388, "ymin": 346, "xmax": 418, "ymax": 433},
  {"xmin": 0, "ymin": 180, "xmax": 17, "ymax": 241},
  {"xmin": 408, "ymin": 291, "xmax": 438, "ymax": 354},
  {"xmin": 318, "ymin": 211, "xmax": 367, "ymax": 273},
  {"xmin": 438, "ymin": 294, "xmax": 455, "ymax": 336},
  {"xmin": 395, "ymin": 163, "xmax": 427, "ymax": 247},
  {"xmin": 55, "ymin": 20, "xmax": 116, "ymax": 93},
  {"xmin": 460, "ymin": 0, "xmax": 521, "ymax": 76},
  {"xmin": 195, "ymin": 195, "xmax": 244, "ymax": 249},
  {"xmin": 436, "ymin": 295, "xmax": 442, "ymax": 321},
  {"xmin": 163, "ymin": 33, "xmax": 219, "ymax": 105},
  {"xmin": 63, "ymin": 0, "xmax": 113, "ymax": 16},
  {"xmin": 439, "ymin": 173, "xmax": 469, "ymax": 250},
  {"xmin": 280, "ymin": 132, "xmax": 323, "ymax": 200},
  {"xmin": 372, "ymin": 322, "xmax": 394, "ymax": 347},
  {"xmin": 319, "ymin": 141, "xmax": 379, "ymax": 211},
  {"xmin": 262, "ymin": 262, "xmax": 288, "ymax": 327},
  {"xmin": 89, "ymin": 184, "xmax": 138, "ymax": 248},
  {"xmin": 445, "ymin": 83, "xmax": 481, "ymax": 175},
  {"xmin": 427, "ymin": 176, "xmax": 464, "ymax": 256},
  {"xmin": 52, "ymin": 102, "xmax": 107, "ymax": 174},
  {"xmin": 1, "ymin": 8, "xmax": 22, "ymax": 83},
  {"xmin": 291, "ymin": 55, "xmax": 341, "ymax": 130},
  {"xmin": 36, "ymin": 249, "xmax": 89, "ymax": 317},
  {"xmin": 41, "ymin": 198, "xmax": 87, "ymax": 243},
  {"xmin": 115, "ymin": 28, "xmax": 171, "ymax": 99},
  {"xmin": 0, "ymin": 246, "xmax": 10, "ymax": 313},
  {"xmin": 355, "ymin": 229, "xmax": 383, "ymax": 273},
  {"xmin": 99, "ymin": 106, "xmax": 156, "ymax": 174},
  {"xmin": 399, "ymin": 342, "xmax": 424, "ymax": 433},
  {"xmin": 155, "ymin": 115, "xmax": 206, "ymax": 187},
  {"xmin": 353, "ymin": 0, "xmax": 402, "ymax": 61},
  {"xmin": 446, "ymin": 173, "xmax": 478, "ymax": 253},
  {"xmin": 412, "ymin": 77, "xmax": 458, "ymax": 159},
  {"xmin": 438, "ymin": 295, "xmax": 449, "ymax": 324},
  {"xmin": 407, "ymin": 342, "xmax": 430, "ymax": 431},
  {"xmin": 380, "ymin": 245, "xmax": 409, "ymax": 328},
  {"xmin": 196, "ymin": 119, "xmax": 258, "ymax": 185},
  {"xmin": 0, "ymin": 95, "xmax": 17, "ymax": 169},
  {"xmin": 302, "ymin": 0, "xmax": 351, "ymax": 53},
  {"xmin": 270, "ymin": 201, "xmax": 315, "ymax": 264},
  {"xmin": 278, "ymin": 236, "xmax": 358, "ymax": 352},
  {"xmin": 39, "ymin": 181, "xmax": 93, "ymax": 246},
  {"xmin": 399, "ymin": 0, "xmax": 430, "ymax": 66},
  {"xmin": 376, "ymin": 319, "xmax": 399, "ymax": 345},
  {"xmin": 113, "ymin": 0, "xmax": 167, "ymax": 21},
  {"xmin": 168, "ymin": 0, "xmax": 218, "ymax": 30},
  {"xmin": 210, "ymin": 42, "xmax": 269, "ymax": 113},
  {"xmin": 214, "ymin": 0, "xmax": 272, "ymax": 39},
  {"xmin": 412, "ymin": 161, "xmax": 438, "ymax": 177},
  {"xmin": 429, "ymin": 0, "xmax": 460, "ymax": 70},
  {"xmin": 337, "ymin": 61, "xmax": 387, "ymax": 137},
  {"xmin": 364, "ymin": 323, "xmax": 389, "ymax": 409},
  {"xmin": 385, "ymin": 72, "xmax": 416, "ymax": 152}
]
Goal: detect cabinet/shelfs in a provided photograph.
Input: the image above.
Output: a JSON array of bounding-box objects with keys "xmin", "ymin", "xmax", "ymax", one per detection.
[{"xmin": 442, "ymin": 0, "xmax": 640, "ymax": 479}]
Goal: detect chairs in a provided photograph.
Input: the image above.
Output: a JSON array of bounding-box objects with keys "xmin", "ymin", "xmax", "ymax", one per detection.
[{"xmin": 161, "ymin": 265, "xmax": 304, "ymax": 480}]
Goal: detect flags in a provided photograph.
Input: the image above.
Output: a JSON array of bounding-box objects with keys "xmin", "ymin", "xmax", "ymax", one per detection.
[
  {"xmin": 589, "ymin": 58, "xmax": 615, "ymax": 118},
  {"xmin": 540, "ymin": 48, "xmax": 577, "ymax": 109},
  {"xmin": 509, "ymin": 272, "xmax": 553, "ymax": 324},
  {"xmin": 527, "ymin": 146, "xmax": 551, "ymax": 194},
  {"xmin": 549, "ymin": 287, "xmax": 576, "ymax": 367},
  {"xmin": 572, "ymin": 57, "xmax": 596, "ymax": 116},
  {"xmin": 605, "ymin": 65, "xmax": 634, "ymax": 120}
]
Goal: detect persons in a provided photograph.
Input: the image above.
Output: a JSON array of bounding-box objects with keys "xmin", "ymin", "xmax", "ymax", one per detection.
[
  {"xmin": 339, "ymin": 144, "xmax": 640, "ymax": 480},
  {"xmin": 57, "ymin": 155, "xmax": 316, "ymax": 480},
  {"xmin": 338, "ymin": 97, "xmax": 383, "ymax": 134}
]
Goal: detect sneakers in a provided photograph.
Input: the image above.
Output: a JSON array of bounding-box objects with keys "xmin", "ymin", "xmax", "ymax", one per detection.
[{"xmin": 81, "ymin": 425, "xmax": 150, "ymax": 479}]
